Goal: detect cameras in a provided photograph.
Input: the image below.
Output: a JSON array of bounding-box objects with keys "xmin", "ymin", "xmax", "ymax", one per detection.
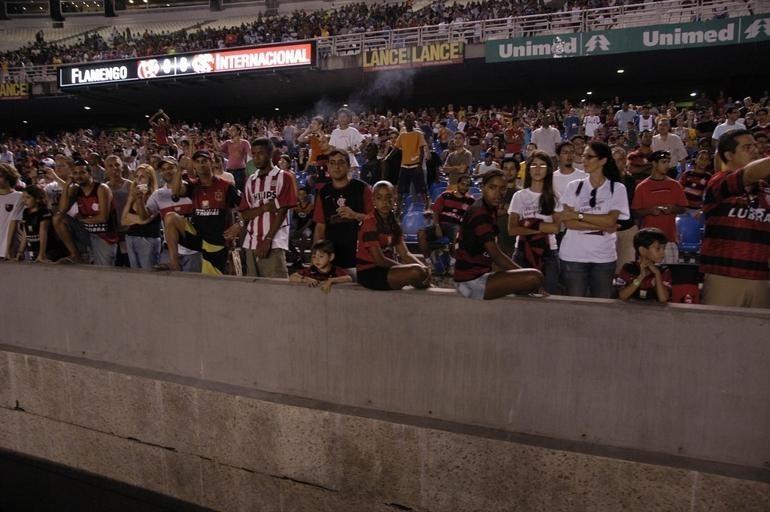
[
  {"xmin": 658, "ymin": 206, "xmax": 669, "ymax": 210},
  {"xmin": 136, "ymin": 183, "xmax": 148, "ymax": 193}
]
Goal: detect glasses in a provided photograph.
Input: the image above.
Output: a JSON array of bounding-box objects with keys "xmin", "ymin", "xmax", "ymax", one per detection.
[
  {"xmin": 589, "ymin": 188, "xmax": 597, "ymax": 208},
  {"xmin": 529, "ymin": 164, "xmax": 548, "ymax": 169},
  {"xmin": 580, "ymin": 154, "xmax": 599, "ymax": 160}
]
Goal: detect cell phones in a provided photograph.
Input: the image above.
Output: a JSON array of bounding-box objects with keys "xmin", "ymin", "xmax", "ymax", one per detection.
[{"xmin": 37, "ymin": 169, "xmax": 46, "ymax": 174}]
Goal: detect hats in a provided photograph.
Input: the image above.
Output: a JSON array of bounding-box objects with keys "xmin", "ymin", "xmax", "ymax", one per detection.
[
  {"xmin": 157, "ymin": 155, "xmax": 178, "ymax": 166},
  {"xmin": 40, "ymin": 157, "xmax": 55, "ymax": 167},
  {"xmin": 191, "ymin": 150, "xmax": 212, "ymax": 161}
]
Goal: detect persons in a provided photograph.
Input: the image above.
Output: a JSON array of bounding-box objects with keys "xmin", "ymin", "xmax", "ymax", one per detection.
[
  {"xmin": 3, "ymin": 88, "xmax": 769, "ymax": 307},
  {"xmin": 1, "ymin": 1, "xmax": 729, "ymax": 80}
]
